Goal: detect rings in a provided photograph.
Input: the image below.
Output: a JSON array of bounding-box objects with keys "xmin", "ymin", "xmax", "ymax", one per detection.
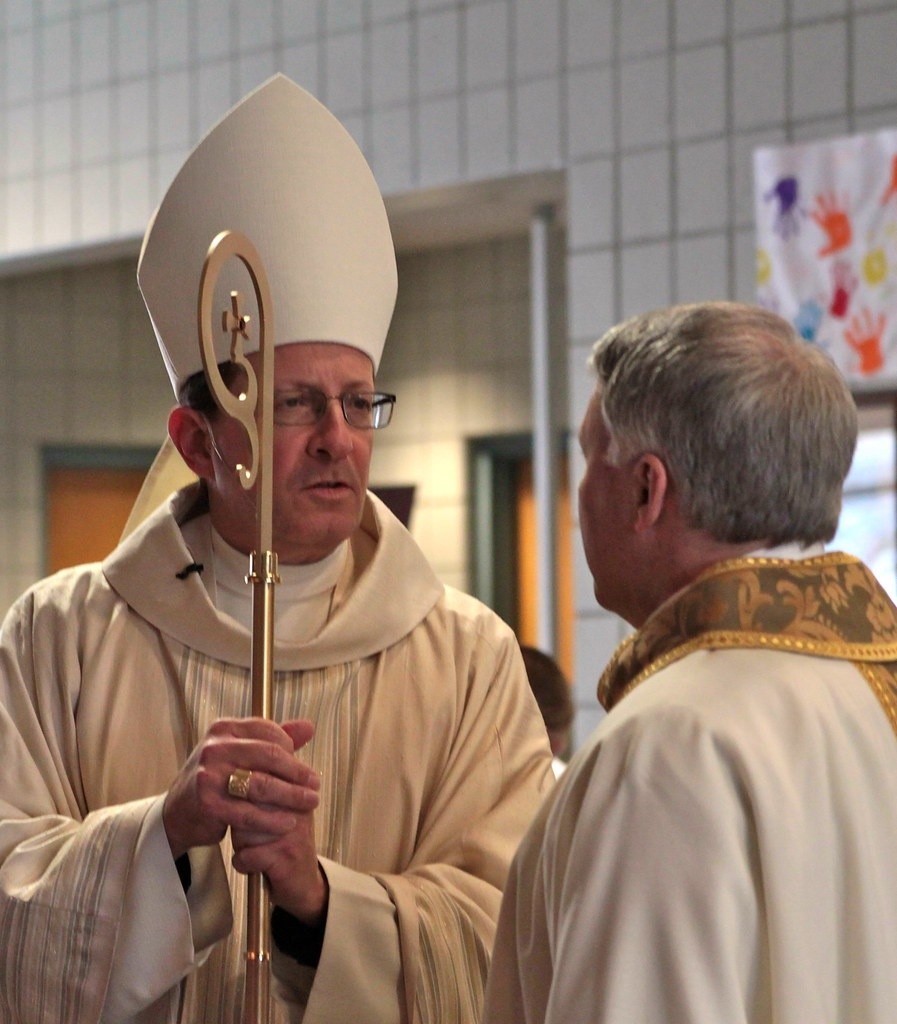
[{"xmin": 227, "ymin": 764, "xmax": 254, "ymax": 799}]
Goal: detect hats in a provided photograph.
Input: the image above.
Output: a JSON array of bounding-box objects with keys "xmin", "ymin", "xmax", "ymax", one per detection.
[{"xmin": 118, "ymin": 72, "xmax": 398, "ymax": 544}]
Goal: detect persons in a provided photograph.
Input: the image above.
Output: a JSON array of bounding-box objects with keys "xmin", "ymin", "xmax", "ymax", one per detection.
[
  {"xmin": 0, "ymin": 73, "xmax": 560, "ymax": 1024},
  {"xmin": 481, "ymin": 303, "xmax": 897, "ymax": 1024}
]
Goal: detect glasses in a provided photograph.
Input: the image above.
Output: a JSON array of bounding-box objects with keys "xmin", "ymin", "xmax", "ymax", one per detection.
[{"xmin": 274, "ymin": 389, "xmax": 397, "ymax": 430}]
